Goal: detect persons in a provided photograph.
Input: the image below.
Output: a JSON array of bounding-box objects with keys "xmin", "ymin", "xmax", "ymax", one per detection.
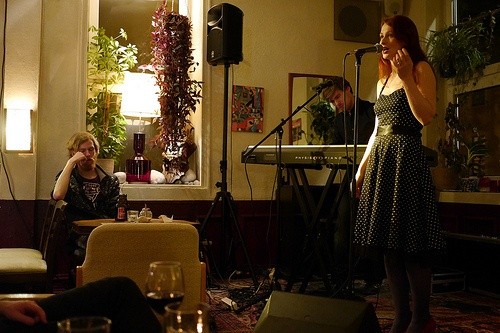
[
  {"xmin": 322, "ymin": 76, "xmax": 386, "ymax": 295},
  {"xmin": 0, "ymin": 276, "xmax": 163, "ymax": 333},
  {"xmin": 51, "ymin": 132, "xmax": 120, "ymax": 266},
  {"xmin": 349, "ymin": 15, "xmax": 447, "ymax": 333}
]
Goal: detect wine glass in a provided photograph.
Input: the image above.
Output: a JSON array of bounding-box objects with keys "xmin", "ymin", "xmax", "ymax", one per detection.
[{"xmin": 142, "ymin": 260, "xmax": 185, "ymax": 333}]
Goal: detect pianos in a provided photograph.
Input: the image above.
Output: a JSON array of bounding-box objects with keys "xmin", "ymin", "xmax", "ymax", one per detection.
[{"xmin": 240, "ymin": 145, "xmax": 375, "ymax": 299}]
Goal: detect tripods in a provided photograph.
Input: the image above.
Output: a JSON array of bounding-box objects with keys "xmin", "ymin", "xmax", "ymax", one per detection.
[{"xmin": 199, "ymin": 65, "xmax": 258, "ymax": 291}]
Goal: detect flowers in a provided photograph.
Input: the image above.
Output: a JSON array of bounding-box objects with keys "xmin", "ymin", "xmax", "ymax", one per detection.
[{"xmin": 99, "ymin": 28, "xmax": 139, "ymax": 75}]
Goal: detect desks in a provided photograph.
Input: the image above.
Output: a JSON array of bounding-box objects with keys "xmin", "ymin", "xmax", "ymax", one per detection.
[{"xmin": 72, "ymin": 219, "xmax": 202, "ymax": 235}]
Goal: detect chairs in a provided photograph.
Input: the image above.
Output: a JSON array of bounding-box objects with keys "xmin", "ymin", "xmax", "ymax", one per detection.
[{"xmin": 0, "ymin": 200, "xmax": 206, "ymax": 302}]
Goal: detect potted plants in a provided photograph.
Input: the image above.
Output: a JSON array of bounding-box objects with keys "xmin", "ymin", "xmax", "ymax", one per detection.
[
  {"xmin": 309, "ymin": 102, "xmax": 336, "ymax": 143},
  {"xmin": 453, "ymin": 136, "xmax": 488, "ymax": 191},
  {"xmin": 420, "ymin": 18, "xmax": 489, "ymax": 87},
  {"xmin": 86, "ymin": 25, "xmax": 138, "ymax": 180},
  {"xmin": 139, "ymin": 0, "xmax": 205, "ymax": 184}
]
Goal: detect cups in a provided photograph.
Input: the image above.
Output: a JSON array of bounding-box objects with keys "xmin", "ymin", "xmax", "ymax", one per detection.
[
  {"xmin": 57, "ymin": 316, "xmax": 112, "ymax": 333},
  {"xmin": 162, "ymin": 301, "xmax": 212, "ymax": 333},
  {"xmin": 126, "ymin": 209, "xmax": 139, "ymax": 222}
]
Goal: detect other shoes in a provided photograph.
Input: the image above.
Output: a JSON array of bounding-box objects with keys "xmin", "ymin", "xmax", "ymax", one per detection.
[{"xmin": 364, "ymin": 280, "xmax": 381, "ymax": 294}]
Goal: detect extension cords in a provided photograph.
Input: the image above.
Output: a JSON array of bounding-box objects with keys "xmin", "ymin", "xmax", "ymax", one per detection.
[{"xmin": 220, "ymin": 297, "xmax": 237, "ymax": 311}]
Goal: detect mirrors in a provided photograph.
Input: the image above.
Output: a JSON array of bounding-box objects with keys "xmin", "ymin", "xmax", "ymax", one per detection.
[{"xmin": 289, "ymin": 73, "xmax": 339, "ymax": 147}]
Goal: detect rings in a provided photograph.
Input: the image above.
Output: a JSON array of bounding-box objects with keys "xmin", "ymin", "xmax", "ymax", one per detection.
[{"xmin": 396, "ymin": 60, "xmax": 400, "ymax": 65}]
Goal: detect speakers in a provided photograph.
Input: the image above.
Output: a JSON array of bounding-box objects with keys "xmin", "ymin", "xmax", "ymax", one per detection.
[
  {"xmin": 207, "ymin": 2, "xmax": 244, "ymax": 66},
  {"xmin": 384, "ymin": 0, "xmax": 403, "ymax": 17},
  {"xmin": 253, "ymin": 291, "xmax": 381, "ymax": 333}
]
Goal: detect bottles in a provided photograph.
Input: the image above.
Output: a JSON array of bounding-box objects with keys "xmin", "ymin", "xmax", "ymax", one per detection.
[
  {"xmin": 139, "ymin": 208, "xmax": 152, "ymax": 220},
  {"xmin": 115, "ymin": 194, "xmax": 128, "ymax": 221}
]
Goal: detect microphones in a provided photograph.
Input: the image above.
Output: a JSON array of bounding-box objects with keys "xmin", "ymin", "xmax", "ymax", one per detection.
[
  {"xmin": 358, "ymin": 44, "xmax": 383, "ymax": 54},
  {"xmin": 315, "ymin": 79, "xmax": 337, "ymax": 91}
]
